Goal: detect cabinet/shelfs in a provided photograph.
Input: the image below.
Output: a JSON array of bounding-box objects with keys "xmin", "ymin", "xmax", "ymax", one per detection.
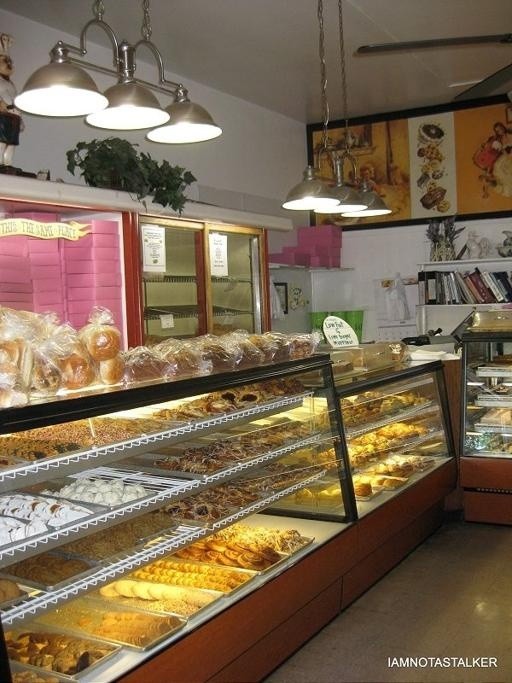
[{"xmin": 0, "ymin": 175, "xmax": 511, "ymax": 682}]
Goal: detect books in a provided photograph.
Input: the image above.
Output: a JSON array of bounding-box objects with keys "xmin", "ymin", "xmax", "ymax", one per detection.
[{"xmin": 418, "ymin": 267, "xmax": 512, "ymax": 305}]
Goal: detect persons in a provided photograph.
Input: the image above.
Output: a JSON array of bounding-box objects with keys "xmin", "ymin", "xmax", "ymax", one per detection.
[
  {"xmin": 0, "ymin": 54, "xmax": 25, "ymax": 166},
  {"xmin": 359, "ymin": 165, "xmax": 383, "ymax": 197},
  {"xmin": 492, "ymin": 122, "xmax": 511, "ymax": 196}
]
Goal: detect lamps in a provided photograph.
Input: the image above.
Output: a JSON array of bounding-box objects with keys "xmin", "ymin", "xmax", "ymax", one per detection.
[
  {"xmin": 280, "ymin": 1, "xmax": 392, "ymax": 219},
  {"xmin": 13, "ymin": 1, "xmax": 224, "ymax": 144}
]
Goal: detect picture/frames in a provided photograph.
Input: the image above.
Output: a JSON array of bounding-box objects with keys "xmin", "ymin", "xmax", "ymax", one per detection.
[{"xmin": 306, "ymin": 93, "xmax": 512, "ymax": 231}]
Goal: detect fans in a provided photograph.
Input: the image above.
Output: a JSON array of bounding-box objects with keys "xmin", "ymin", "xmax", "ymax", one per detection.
[{"xmin": 358, "ymin": 34, "xmax": 512, "ymax": 100}]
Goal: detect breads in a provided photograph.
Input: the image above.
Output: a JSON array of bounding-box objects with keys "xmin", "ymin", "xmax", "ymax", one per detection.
[{"xmin": 0, "ymin": 308, "xmax": 434, "ymax": 683}]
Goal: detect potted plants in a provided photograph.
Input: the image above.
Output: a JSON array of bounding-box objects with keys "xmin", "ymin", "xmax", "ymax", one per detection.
[{"xmin": 66, "ymin": 137, "xmax": 197, "ymax": 217}]
